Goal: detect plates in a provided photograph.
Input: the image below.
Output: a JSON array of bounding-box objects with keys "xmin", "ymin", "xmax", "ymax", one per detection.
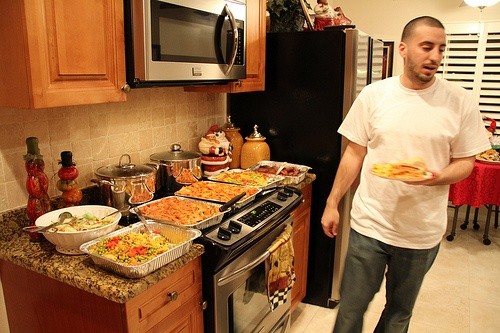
[
  {"xmin": 476, "ymin": 154, "xmax": 500, "ymax": 164},
  {"xmin": 367, "ymin": 164, "xmax": 437, "ymax": 183}
]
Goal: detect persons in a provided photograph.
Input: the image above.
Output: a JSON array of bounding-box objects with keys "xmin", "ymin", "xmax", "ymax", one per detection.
[{"xmin": 321, "ymin": 17, "xmax": 492, "ymax": 333}]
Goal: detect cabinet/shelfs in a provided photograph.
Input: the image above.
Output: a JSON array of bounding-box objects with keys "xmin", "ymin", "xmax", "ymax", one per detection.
[
  {"xmin": 184, "ymin": 0, "xmax": 267, "ymax": 92},
  {"xmin": 0, "ymin": 256, "xmax": 208, "ymax": 333},
  {"xmin": 290, "ymin": 182, "xmax": 313, "ymax": 312},
  {"xmin": 0, "ymin": 0, "xmax": 130, "ymax": 110}
]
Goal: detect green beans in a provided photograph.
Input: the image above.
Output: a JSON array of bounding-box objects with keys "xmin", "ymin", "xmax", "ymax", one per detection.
[{"xmin": 96, "ymin": 240, "xmax": 130, "ymax": 263}]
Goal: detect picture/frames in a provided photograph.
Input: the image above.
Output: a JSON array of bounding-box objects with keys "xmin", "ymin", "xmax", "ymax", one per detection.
[{"xmin": 382, "ymin": 42, "xmax": 394, "ymax": 79}]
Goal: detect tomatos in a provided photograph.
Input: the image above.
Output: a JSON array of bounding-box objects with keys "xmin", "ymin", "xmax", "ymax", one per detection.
[{"xmin": 106, "ymin": 237, "xmax": 148, "ymax": 257}]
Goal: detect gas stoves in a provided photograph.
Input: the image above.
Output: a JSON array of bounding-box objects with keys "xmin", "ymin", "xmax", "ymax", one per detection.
[{"xmin": 195, "ymin": 185, "xmax": 305, "ymax": 271}]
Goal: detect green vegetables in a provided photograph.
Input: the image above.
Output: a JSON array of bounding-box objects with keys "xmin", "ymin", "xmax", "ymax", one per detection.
[{"xmin": 75, "ymin": 213, "xmax": 109, "ymax": 228}]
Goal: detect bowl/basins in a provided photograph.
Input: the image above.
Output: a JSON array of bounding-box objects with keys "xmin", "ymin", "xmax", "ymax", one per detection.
[
  {"xmin": 34, "ymin": 205, "xmax": 122, "ymax": 253},
  {"xmin": 79, "ymin": 160, "xmax": 312, "ymax": 279}
]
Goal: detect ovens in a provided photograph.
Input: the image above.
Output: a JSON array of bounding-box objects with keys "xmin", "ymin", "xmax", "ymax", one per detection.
[{"xmin": 200, "ymin": 214, "xmax": 294, "ymax": 333}]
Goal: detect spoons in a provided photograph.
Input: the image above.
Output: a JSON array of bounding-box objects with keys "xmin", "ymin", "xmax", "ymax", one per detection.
[{"xmin": 35, "ymin": 212, "xmax": 72, "ymax": 234}]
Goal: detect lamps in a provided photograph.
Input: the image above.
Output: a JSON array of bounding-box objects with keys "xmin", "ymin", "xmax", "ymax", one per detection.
[{"xmin": 463, "ymin": 0, "xmax": 499, "ymax": 12}]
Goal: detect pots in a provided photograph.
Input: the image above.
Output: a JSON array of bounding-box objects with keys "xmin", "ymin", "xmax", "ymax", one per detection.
[
  {"xmin": 90, "ymin": 153, "xmax": 159, "ymax": 217},
  {"xmin": 149, "ymin": 144, "xmax": 201, "ymax": 194}
]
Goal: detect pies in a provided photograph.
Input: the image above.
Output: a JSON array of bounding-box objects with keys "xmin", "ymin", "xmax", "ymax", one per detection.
[{"xmin": 372, "ymin": 163, "xmax": 423, "ymax": 179}]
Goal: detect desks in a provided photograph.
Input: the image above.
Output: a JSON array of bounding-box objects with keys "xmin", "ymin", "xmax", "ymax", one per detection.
[{"xmin": 447, "ymin": 161, "xmax": 500, "ymax": 245}]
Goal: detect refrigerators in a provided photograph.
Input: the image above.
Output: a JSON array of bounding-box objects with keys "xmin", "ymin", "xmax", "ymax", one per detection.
[{"xmin": 226, "ymin": 24, "xmax": 385, "ymax": 309}]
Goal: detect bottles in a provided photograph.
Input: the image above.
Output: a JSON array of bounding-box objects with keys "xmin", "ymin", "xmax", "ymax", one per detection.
[
  {"xmin": 240, "ymin": 124, "xmax": 272, "ymax": 169},
  {"xmin": 222, "ymin": 127, "xmax": 244, "ymax": 169}
]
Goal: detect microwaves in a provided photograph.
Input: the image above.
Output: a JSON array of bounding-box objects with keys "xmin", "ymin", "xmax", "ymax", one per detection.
[{"xmin": 122, "ymin": 0, "xmax": 248, "ymax": 89}]
